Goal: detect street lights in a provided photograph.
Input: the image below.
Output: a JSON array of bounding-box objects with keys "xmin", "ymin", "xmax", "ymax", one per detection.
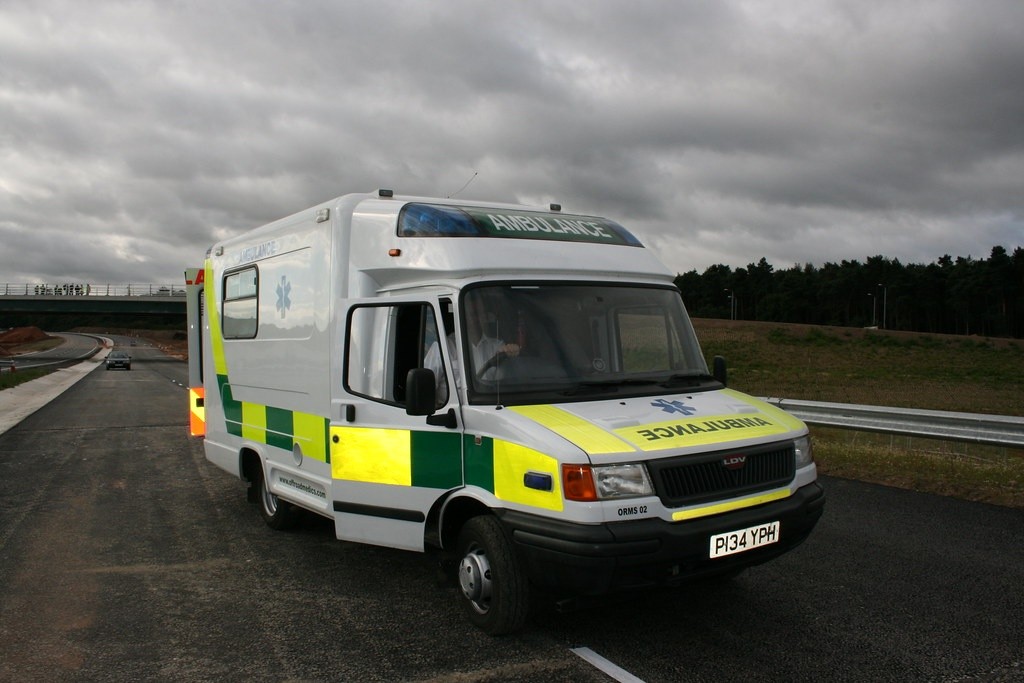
[
  {"xmin": 879, "ymin": 283, "xmax": 887, "ymax": 330},
  {"xmin": 723, "ymin": 288, "xmax": 733, "ymax": 321},
  {"xmin": 728, "ymin": 295, "xmax": 737, "ymax": 321}
]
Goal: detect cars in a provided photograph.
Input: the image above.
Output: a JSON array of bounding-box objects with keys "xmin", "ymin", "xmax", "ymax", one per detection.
[{"xmin": 104, "ymin": 350, "xmax": 132, "ymax": 371}]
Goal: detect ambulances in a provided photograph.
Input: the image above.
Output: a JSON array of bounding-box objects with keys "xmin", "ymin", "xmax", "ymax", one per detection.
[{"xmin": 185, "ymin": 187, "xmax": 829, "ymax": 636}]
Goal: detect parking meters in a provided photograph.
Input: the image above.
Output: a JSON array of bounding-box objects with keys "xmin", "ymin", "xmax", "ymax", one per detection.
[{"xmin": 868, "ymin": 294, "xmax": 876, "ymax": 326}]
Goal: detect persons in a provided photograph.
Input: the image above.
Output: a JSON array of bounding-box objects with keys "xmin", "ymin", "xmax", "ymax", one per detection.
[
  {"xmin": 424, "ymin": 290, "xmax": 523, "ymax": 409},
  {"xmin": 34, "ymin": 283, "xmax": 91, "ymax": 296}
]
